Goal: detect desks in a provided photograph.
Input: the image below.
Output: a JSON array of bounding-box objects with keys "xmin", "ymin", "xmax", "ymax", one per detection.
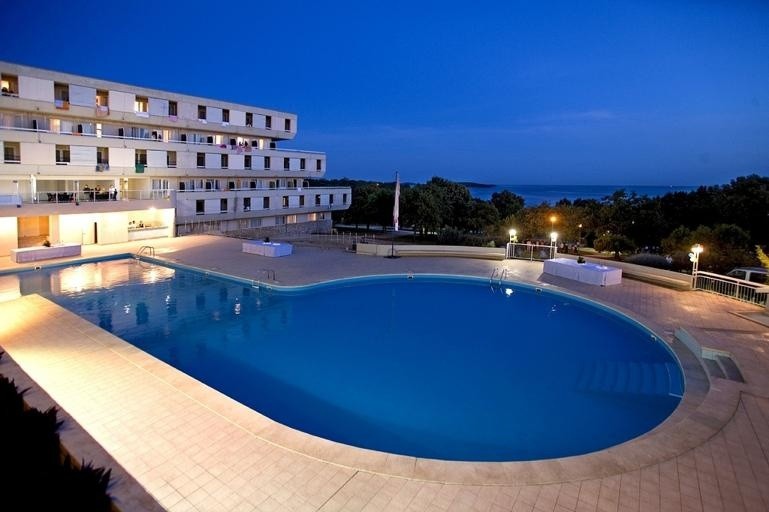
[
  {"xmin": 242, "ymin": 242, "xmax": 293, "ymax": 257},
  {"xmin": 8, "ymin": 243, "xmax": 80, "ymax": 263},
  {"xmin": 543, "ymin": 259, "xmax": 623, "ymax": 284}
]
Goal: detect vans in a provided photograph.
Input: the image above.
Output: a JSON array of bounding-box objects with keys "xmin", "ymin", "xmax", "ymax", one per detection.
[{"xmin": 726, "ymin": 265, "xmax": 769, "ymax": 285}]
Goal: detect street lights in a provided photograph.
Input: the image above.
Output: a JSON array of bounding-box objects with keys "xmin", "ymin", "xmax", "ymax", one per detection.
[
  {"xmin": 549, "ymin": 217, "xmax": 556, "ymax": 231},
  {"xmin": 688, "ymin": 243, "xmax": 703, "ymax": 289},
  {"xmin": 508, "ymin": 229, "xmax": 517, "ymax": 242},
  {"xmin": 550, "ymin": 232, "xmax": 558, "ymax": 258}
]
